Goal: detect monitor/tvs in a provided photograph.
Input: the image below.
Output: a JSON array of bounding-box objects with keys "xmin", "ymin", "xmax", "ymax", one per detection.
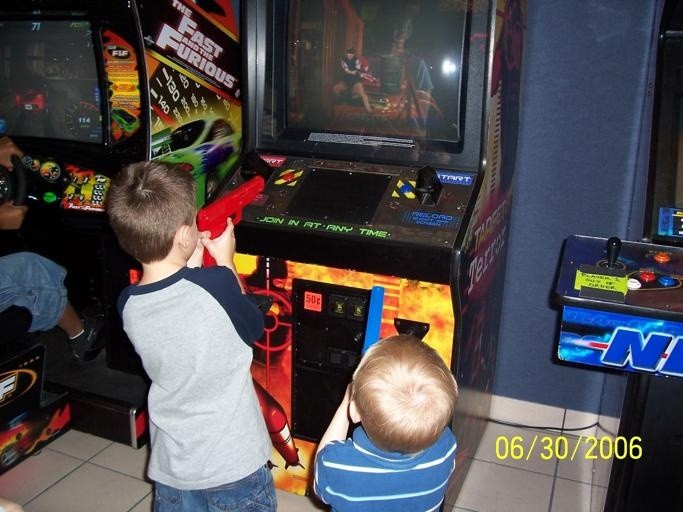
[
  {"xmin": 241, "ymin": 2, "xmax": 495, "ymax": 176},
  {"xmin": 0, "ymin": 0, "xmax": 113, "ymax": 153},
  {"xmin": 644, "ymin": 3, "xmax": 683, "ymax": 246}
]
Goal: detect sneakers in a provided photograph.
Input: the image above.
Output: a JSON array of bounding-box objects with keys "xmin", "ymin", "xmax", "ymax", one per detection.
[{"xmin": 68, "ymin": 305, "xmax": 115, "ymax": 363}]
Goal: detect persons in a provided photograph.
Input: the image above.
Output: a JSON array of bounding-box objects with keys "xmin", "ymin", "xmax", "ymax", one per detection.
[
  {"xmin": 333, "ymin": 46, "xmax": 376, "ymax": 116},
  {"xmin": 314, "ymin": 335, "xmax": 457, "ymax": 512},
  {"xmin": 0, "ymin": 134, "xmax": 111, "ymax": 361},
  {"xmin": 358, "ymin": 13, "xmax": 434, "ymax": 138},
  {"xmin": 105, "ymin": 161, "xmax": 276, "ymax": 512}
]
